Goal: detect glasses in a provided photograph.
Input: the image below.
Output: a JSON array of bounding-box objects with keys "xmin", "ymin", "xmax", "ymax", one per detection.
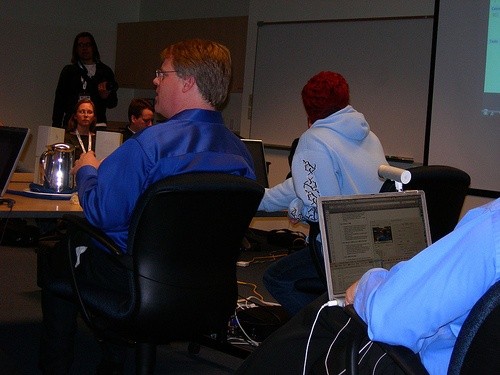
[{"xmin": 155, "ymin": 68, "xmax": 182, "ymax": 79}]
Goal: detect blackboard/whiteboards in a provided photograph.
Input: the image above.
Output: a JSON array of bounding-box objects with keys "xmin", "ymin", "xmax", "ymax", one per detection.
[{"xmin": 247, "ymin": 15, "xmax": 435, "ymax": 166}]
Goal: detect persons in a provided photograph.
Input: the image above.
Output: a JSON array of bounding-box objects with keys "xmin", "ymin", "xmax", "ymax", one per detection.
[
  {"xmin": 64, "ymin": 100, "xmax": 98, "ymax": 160},
  {"xmin": 122, "ymin": 98, "xmax": 154, "ymax": 145},
  {"xmin": 232, "ymin": 196, "xmax": 499, "ymax": 374},
  {"xmin": 260, "ymin": 71, "xmax": 393, "ymax": 316},
  {"xmin": 52, "ymin": 33, "xmax": 118, "ymax": 131},
  {"xmin": 42, "ymin": 39, "xmax": 258, "ymax": 373}
]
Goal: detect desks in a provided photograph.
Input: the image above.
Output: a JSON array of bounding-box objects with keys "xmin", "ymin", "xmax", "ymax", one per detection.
[{"xmin": 0, "ymin": 173, "xmax": 311, "ymax": 238}]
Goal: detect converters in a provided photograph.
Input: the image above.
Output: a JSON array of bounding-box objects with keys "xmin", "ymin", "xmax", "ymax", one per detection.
[{"xmin": 237, "ymin": 261, "xmax": 249, "ymax": 267}]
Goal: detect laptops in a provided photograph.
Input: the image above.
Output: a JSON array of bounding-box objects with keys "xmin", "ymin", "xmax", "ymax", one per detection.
[
  {"xmin": 318, "ymin": 189, "xmax": 432, "ymax": 328},
  {"xmin": 1, "ymin": 125, "xmax": 31, "ymax": 197},
  {"xmin": 240, "ymin": 138, "xmax": 270, "ymax": 189}
]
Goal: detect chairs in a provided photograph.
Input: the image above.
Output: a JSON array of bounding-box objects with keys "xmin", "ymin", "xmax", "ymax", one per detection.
[
  {"xmin": 344, "ymin": 281, "xmax": 500, "ymax": 375},
  {"xmin": 67, "ymin": 174, "xmax": 266, "ymax": 375},
  {"xmin": 310, "ymin": 164, "xmax": 471, "ymax": 280}
]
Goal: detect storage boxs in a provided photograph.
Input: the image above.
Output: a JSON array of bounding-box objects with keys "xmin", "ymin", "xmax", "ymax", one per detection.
[{"xmin": 34, "ymin": 126, "xmax": 65, "ymax": 186}]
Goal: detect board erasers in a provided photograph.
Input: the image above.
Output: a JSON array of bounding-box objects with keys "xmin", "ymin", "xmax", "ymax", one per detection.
[{"xmin": 386, "ymin": 154, "xmax": 414, "ymax": 162}]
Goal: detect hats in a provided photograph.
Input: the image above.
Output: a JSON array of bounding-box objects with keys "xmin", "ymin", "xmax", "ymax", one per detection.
[{"xmin": 301, "ymin": 71, "xmax": 350, "ymax": 121}]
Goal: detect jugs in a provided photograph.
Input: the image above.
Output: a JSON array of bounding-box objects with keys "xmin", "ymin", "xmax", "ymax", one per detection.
[{"xmin": 39, "ymin": 143, "xmax": 76, "ymax": 193}]
